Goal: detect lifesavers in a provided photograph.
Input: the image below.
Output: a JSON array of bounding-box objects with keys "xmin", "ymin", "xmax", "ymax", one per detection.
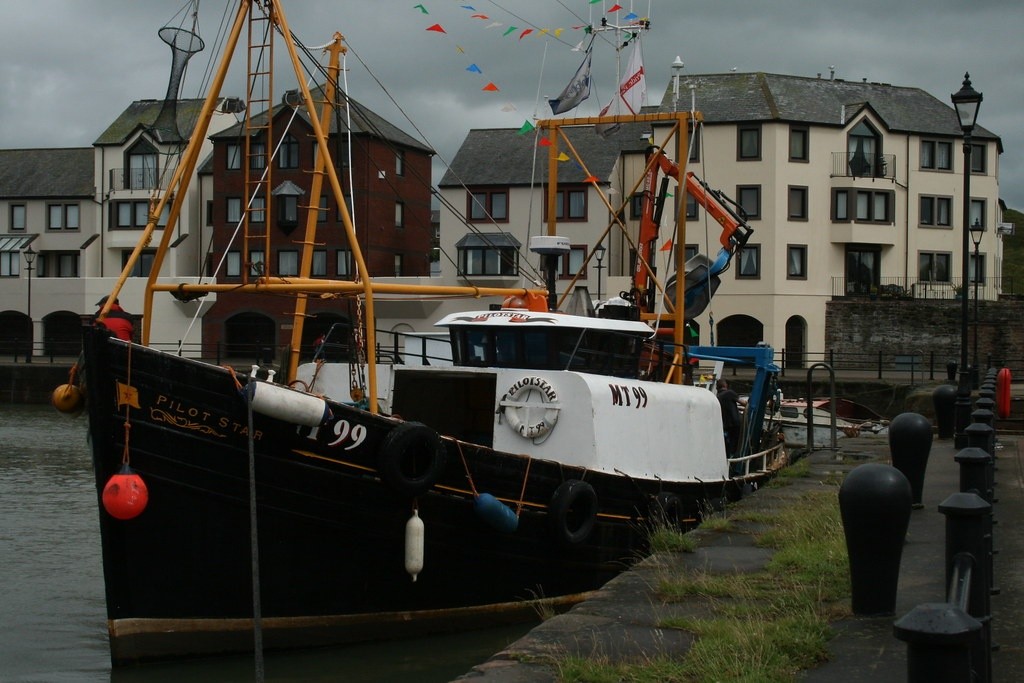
[
  {"xmin": 388, "ymin": 422, "xmax": 439, "ymax": 487},
  {"xmin": 503, "ymin": 380, "xmax": 557, "ymax": 437},
  {"xmin": 547, "ymin": 483, "xmax": 599, "ymax": 543},
  {"xmin": 648, "ymin": 493, "xmax": 681, "ymax": 537}
]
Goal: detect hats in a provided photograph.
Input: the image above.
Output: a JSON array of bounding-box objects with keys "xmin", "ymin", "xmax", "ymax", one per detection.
[{"xmin": 95, "ymin": 296, "xmax": 119, "ymax": 306}]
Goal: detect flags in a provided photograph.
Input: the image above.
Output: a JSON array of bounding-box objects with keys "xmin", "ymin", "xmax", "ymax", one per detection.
[
  {"xmin": 549, "ymin": 32, "xmax": 592, "ymax": 116},
  {"xmin": 598, "ymin": 29, "xmax": 647, "ymax": 118}
]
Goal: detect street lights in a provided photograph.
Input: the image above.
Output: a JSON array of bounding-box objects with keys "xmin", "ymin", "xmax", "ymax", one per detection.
[
  {"xmin": 22, "ymin": 243, "xmax": 37, "ymax": 363},
  {"xmin": 592, "ymin": 243, "xmax": 607, "ymax": 299},
  {"xmin": 948, "ymin": 70, "xmax": 985, "ymax": 453}
]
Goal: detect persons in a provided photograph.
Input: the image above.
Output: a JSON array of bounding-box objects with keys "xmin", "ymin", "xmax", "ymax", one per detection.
[
  {"xmin": 90, "ymin": 294, "xmax": 135, "ymax": 343},
  {"xmin": 716, "ymin": 379, "xmax": 748, "ymax": 459}
]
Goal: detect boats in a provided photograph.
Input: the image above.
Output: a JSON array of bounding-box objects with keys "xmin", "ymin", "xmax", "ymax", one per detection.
[
  {"xmin": 736, "ymin": 393, "xmax": 888, "ymax": 448},
  {"xmin": 53, "ymin": 0, "xmax": 793, "ymax": 682}
]
